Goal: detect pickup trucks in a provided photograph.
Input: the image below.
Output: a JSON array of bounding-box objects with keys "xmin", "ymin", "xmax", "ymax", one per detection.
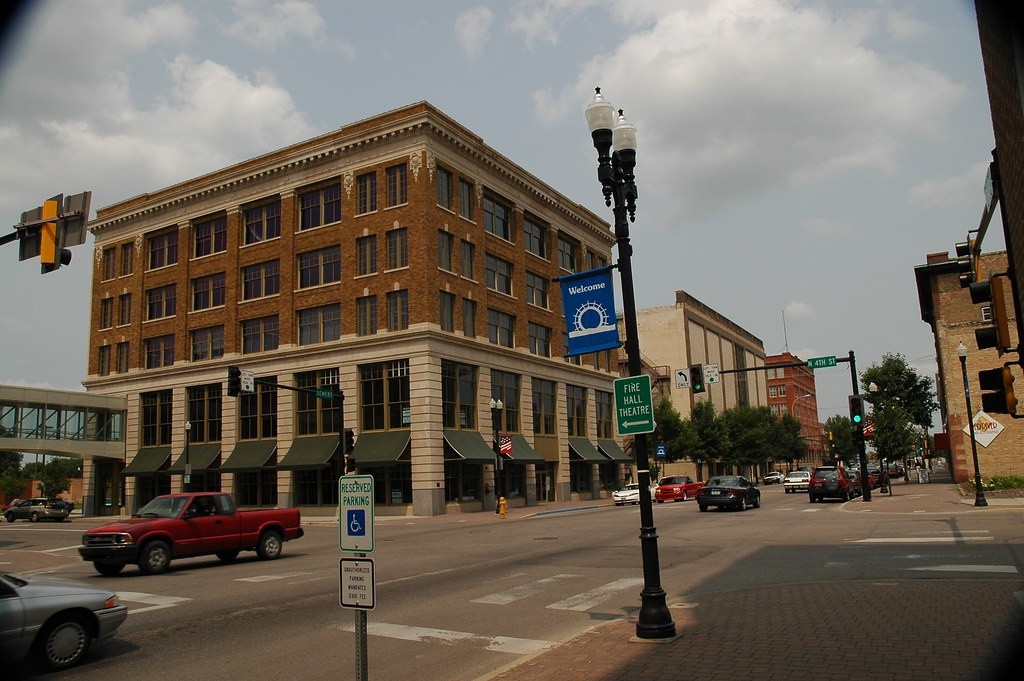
[
  {"xmin": 79, "ymin": 491, "xmax": 304, "ymax": 573},
  {"xmin": 655, "ymin": 475, "xmax": 706, "ymax": 504}
]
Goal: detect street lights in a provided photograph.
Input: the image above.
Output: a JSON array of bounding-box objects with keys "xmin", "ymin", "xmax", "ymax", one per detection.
[
  {"xmin": 790, "ymin": 394, "xmax": 814, "ymax": 422},
  {"xmin": 584, "ymin": 83, "xmax": 679, "ymax": 636},
  {"xmin": 489, "ymin": 398, "xmax": 503, "ymax": 515},
  {"xmin": 184, "ymin": 420, "xmax": 192, "ymax": 493},
  {"xmin": 957, "ymin": 340, "xmax": 988, "ymax": 507}
]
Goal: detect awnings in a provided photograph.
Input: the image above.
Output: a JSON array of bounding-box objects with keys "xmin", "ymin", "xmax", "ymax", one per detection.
[
  {"xmin": 568, "ymin": 438, "xmax": 608, "ymax": 463},
  {"xmin": 597, "ymin": 439, "xmax": 633, "ymax": 463},
  {"xmin": 119, "ymin": 446, "xmax": 171, "ymax": 476},
  {"xmin": 436, "ymin": 430, "xmax": 497, "ymax": 465},
  {"xmin": 167, "ymin": 443, "xmax": 221, "ymax": 474},
  {"xmin": 218, "ymin": 440, "xmax": 276, "ymax": 471},
  {"xmin": 349, "ymin": 430, "xmax": 411, "ymax": 467},
  {"xmin": 492, "ymin": 433, "xmax": 545, "ymax": 464},
  {"xmin": 277, "ymin": 435, "xmax": 341, "ymax": 470}
]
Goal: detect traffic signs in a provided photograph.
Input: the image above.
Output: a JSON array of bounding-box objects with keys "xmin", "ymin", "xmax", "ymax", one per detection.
[{"xmin": 675, "ymin": 369, "xmax": 692, "ymax": 389}]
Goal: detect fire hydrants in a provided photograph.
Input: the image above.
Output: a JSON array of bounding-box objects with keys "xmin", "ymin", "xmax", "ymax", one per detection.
[{"xmin": 499, "ymin": 497, "xmax": 506, "ymax": 519}]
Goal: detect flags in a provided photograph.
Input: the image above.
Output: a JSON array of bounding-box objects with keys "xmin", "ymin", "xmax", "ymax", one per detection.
[{"xmin": 499, "ymin": 434, "xmax": 512, "ymax": 455}]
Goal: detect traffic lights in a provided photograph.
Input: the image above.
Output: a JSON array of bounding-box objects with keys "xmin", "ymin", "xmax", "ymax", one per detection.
[
  {"xmin": 342, "ymin": 428, "xmax": 354, "ymax": 455},
  {"xmin": 848, "ymin": 394, "xmax": 865, "ymax": 427},
  {"xmin": 227, "ymin": 365, "xmax": 241, "ymax": 397},
  {"xmin": 688, "ymin": 364, "xmax": 706, "ymax": 394}
]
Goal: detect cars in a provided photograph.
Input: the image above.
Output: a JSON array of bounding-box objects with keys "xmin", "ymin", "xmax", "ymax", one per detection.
[
  {"xmin": 764, "ymin": 472, "xmax": 785, "ymax": 485},
  {"xmin": 810, "ymin": 464, "xmax": 905, "ymax": 503},
  {"xmin": 0, "ymin": 570, "xmax": 132, "ymax": 670},
  {"xmin": 783, "ymin": 471, "xmax": 812, "ymax": 492},
  {"xmin": 612, "ymin": 482, "xmax": 656, "ymax": 506},
  {"xmin": 695, "ymin": 475, "xmax": 760, "ymax": 512},
  {"xmin": 5, "ymin": 497, "xmax": 73, "ymax": 521}
]
